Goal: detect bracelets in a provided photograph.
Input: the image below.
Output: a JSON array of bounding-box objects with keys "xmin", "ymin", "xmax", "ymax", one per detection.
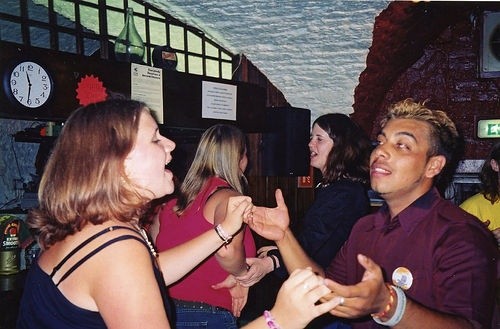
[
  {"xmin": 372, "ymin": 285, "xmax": 406, "ymax": 327},
  {"xmin": 215, "ymin": 224, "xmax": 232, "ymax": 243},
  {"xmin": 265, "ymin": 310, "xmax": 278, "ymax": 329}
]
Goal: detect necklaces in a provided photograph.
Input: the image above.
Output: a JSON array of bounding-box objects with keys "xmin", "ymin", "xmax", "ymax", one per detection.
[{"xmin": 130, "ymin": 218, "xmax": 157, "ymax": 257}]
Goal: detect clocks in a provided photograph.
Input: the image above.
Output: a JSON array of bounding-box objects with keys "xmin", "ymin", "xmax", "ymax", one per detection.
[{"xmin": 3, "ymin": 56, "xmax": 56, "ymax": 114}]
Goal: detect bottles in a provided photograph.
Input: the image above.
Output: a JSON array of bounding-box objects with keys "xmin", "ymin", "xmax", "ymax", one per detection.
[
  {"xmin": 45, "ymin": 122, "xmax": 55, "ymax": 136},
  {"xmin": 114, "ymin": 8, "xmax": 145, "ymax": 63}
]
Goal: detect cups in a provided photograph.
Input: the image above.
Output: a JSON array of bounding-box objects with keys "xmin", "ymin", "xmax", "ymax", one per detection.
[{"xmin": 53, "ymin": 125, "xmax": 63, "ymax": 135}]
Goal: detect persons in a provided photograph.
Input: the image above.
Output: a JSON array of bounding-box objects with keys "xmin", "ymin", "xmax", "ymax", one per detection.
[
  {"xmin": 18, "ymin": 99, "xmax": 344, "ymax": 329},
  {"xmin": 460, "ymin": 156, "xmax": 500, "ymax": 240},
  {"xmin": 235, "ymin": 113, "xmax": 371, "ymax": 329},
  {"xmin": 147, "ymin": 124, "xmax": 256, "ymax": 329},
  {"xmin": 247, "ymin": 98, "xmax": 500, "ymax": 329}
]
